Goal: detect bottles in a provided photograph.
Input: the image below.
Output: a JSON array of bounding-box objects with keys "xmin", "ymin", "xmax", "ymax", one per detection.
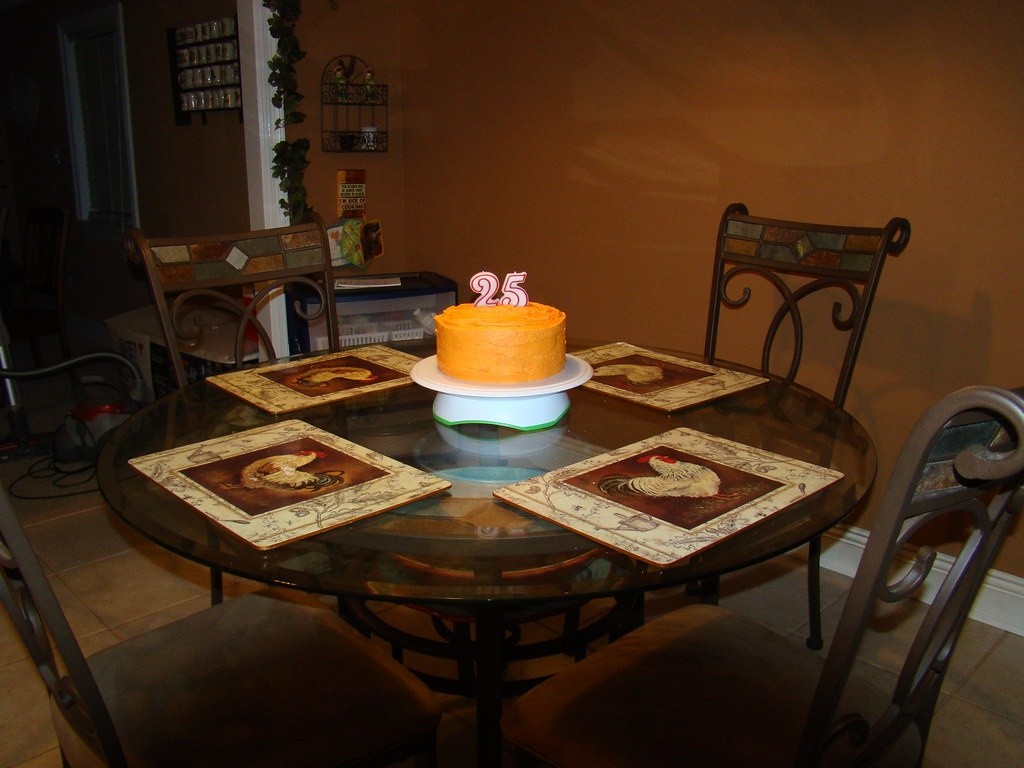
[
  {"xmin": 335, "ymin": 71, "xmax": 348, "ymax": 98},
  {"xmin": 363, "ymin": 72, "xmax": 376, "ymax": 98}
]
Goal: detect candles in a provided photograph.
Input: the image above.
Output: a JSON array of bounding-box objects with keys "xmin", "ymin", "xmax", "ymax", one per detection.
[{"xmin": 469, "ymin": 269, "xmax": 529, "ymax": 308}]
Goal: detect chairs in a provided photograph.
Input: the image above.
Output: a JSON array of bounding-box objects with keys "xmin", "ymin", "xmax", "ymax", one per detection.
[{"xmin": 0, "ymin": 200, "xmax": 1024, "ymax": 768}]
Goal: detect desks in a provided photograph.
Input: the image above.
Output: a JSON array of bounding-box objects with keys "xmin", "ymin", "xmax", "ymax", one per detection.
[{"xmin": 93, "ymin": 330, "xmax": 880, "ymax": 768}]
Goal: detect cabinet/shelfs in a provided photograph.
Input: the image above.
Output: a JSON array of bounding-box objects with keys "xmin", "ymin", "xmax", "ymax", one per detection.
[{"xmin": 165, "ymin": 13, "xmax": 244, "ymax": 128}]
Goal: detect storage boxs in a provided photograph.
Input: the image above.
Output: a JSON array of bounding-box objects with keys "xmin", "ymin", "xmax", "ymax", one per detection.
[
  {"xmin": 103, "ymin": 299, "xmax": 261, "ymax": 403},
  {"xmin": 285, "ymin": 272, "xmax": 459, "ymax": 355}
]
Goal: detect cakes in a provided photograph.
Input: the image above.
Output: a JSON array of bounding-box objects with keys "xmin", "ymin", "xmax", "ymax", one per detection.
[{"xmin": 433, "ymin": 299, "xmax": 567, "ymax": 384}]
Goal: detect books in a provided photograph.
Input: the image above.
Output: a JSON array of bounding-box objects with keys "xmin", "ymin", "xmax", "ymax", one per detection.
[{"xmin": 334, "ymin": 277, "xmax": 401, "ymax": 290}]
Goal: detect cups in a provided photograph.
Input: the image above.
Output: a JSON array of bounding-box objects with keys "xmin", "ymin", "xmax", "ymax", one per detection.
[
  {"xmin": 176, "ymin": 17, "xmax": 241, "ymax": 110},
  {"xmin": 338, "ymin": 135, "xmax": 358, "ymax": 150},
  {"xmin": 361, "ymin": 127, "xmax": 376, "ymax": 149}
]
